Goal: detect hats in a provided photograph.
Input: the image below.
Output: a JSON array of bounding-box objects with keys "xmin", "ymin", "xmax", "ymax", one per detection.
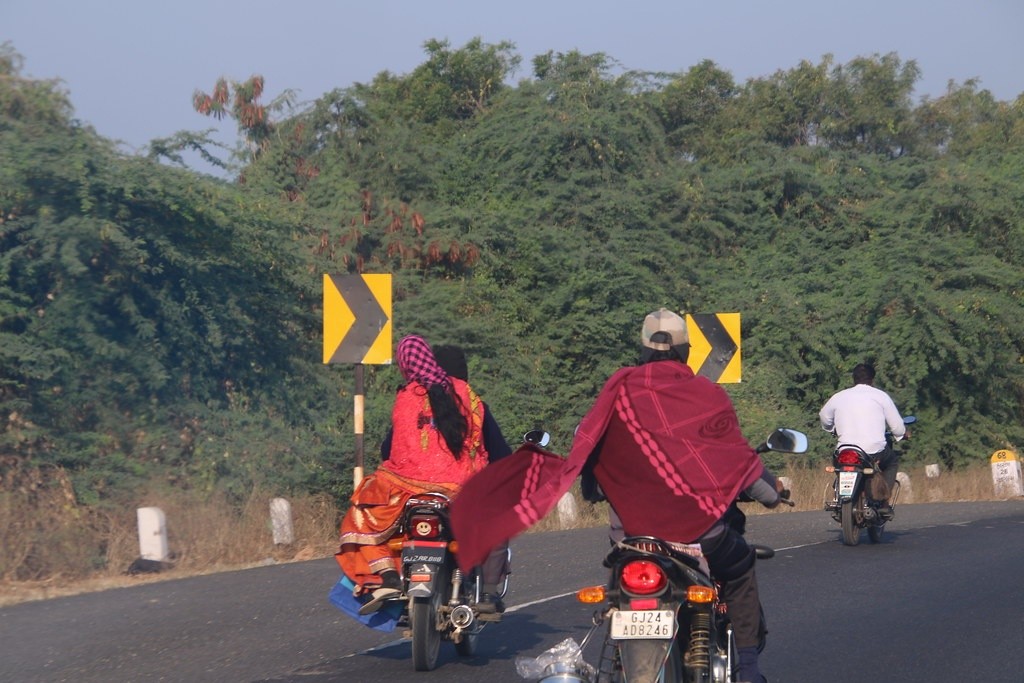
[
  {"xmin": 641, "ymin": 307, "xmax": 688, "ymax": 351},
  {"xmin": 769, "ymin": 430, "xmax": 797, "ymax": 450}
]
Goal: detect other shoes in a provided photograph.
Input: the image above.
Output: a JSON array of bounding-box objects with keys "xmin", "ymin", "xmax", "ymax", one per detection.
[
  {"xmin": 478, "ymin": 592, "xmax": 506, "ymax": 613},
  {"xmin": 879, "ymin": 503, "xmax": 894, "ymax": 520}
]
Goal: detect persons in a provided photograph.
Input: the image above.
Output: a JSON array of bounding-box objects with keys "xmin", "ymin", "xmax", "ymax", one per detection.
[
  {"xmin": 578, "ymin": 309, "xmax": 785, "ymax": 682},
  {"xmin": 819, "ymin": 365, "xmax": 911, "ymax": 519},
  {"xmin": 341, "ymin": 337, "xmax": 511, "ymax": 616}
]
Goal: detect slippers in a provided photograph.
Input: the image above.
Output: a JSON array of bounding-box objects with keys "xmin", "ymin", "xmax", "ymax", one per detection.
[{"xmin": 358, "ymin": 589, "xmax": 404, "ymax": 614}]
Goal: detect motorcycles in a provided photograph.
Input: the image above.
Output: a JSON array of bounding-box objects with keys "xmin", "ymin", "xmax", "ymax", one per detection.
[
  {"xmin": 382, "ymin": 428, "xmax": 554, "ymax": 673},
  {"xmin": 821, "ymin": 415, "xmax": 918, "ymax": 548},
  {"xmin": 537, "ymin": 426, "xmax": 811, "ymax": 683}
]
